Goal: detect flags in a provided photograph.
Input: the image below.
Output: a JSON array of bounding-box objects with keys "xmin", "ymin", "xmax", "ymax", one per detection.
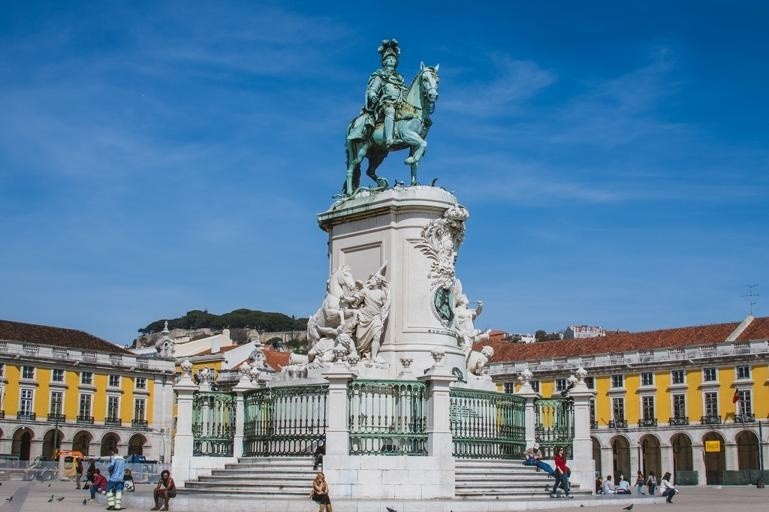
[{"xmin": 732, "ymin": 388, "xmax": 740, "ymax": 403}]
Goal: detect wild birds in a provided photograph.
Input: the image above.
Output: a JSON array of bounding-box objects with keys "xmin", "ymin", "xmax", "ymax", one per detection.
[
  {"xmin": 5, "ymin": 497, "xmax": 13, "ymax": 501},
  {"xmin": 48, "ymin": 495, "xmax": 54, "ymax": 503},
  {"xmin": 624, "ymin": 504, "xmax": 633, "ymax": 510},
  {"xmin": 83, "ymin": 499, "xmax": 87, "ymax": 505},
  {"xmin": 56, "ymin": 497, "xmax": 64, "ymax": 502},
  {"xmin": 387, "ymin": 507, "xmax": 397, "ymax": 512}
]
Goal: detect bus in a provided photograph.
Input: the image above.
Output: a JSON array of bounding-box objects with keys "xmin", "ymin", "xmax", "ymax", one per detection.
[
  {"xmin": 55, "ymin": 450, "xmax": 83, "ymax": 479},
  {"xmin": 84, "ymin": 455, "xmax": 125, "ymax": 472}
]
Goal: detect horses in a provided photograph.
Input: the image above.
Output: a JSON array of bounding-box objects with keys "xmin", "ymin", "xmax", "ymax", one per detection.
[
  {"xmin": 342, "ymin": 61, "xmax": 439, "ymax": 200},
  {"xmin": 307, "ymin": 265, "xmax": 355, "ymax": 349}
]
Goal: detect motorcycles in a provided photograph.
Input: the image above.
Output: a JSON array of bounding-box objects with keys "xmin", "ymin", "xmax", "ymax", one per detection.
[{"xmin": 25, "ymin": 463, "xmax": 52, "ymax": 480}]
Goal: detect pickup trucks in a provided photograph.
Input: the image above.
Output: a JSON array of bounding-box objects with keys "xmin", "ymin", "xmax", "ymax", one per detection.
[{"xmin": 124, "ymin": 456, "xmax": 161, "ymax": 471}]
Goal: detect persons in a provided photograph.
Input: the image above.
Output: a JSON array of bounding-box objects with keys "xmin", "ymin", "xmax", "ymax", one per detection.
[
  {"xmin": 366, "ymin": 38, "xmax": 410, "ymax": 146},
  {"xmin": 660, "ymin": 472, "xmax": 679, "ymax": 504},
  {"xmin": 152, "ymin": 470, "xmax": 176, "ymax": 511},
  {"xmin": 122, "ymin": 468, "xmax": 135, "ymax": 492},
  {"xmin": 89, "ymin": 469, "xmax": 108, "ymax": 501},
  {"xmin": 596, "ymin": 469, "xmax": 656, "ymax": 496},
  {"xmin": 105, "ymin": 448, "xmax": 125, "ymax": 510},
  {"xmin": 87, "ymin": 458, "xmax": 95, "ymax": 483},
  {"xmin": 310, "ymin": 272, "xmax": 392, "ymax": 364},
  {"xmin": 466, "ymin": 345, "xmax": 495, "ymax": 376},
  {"xmin": 550, "ymin": 446, "xmax": 573, "ymax": 498},
  {"xmin": 312, "ymin": 473, "xmax": 333, "ymax": 512},
  {"xmin": 313, "ymin": 444, "xmax": 326, "ymax": 469},
  {"xmin": 452, "ymin": 294, "xmax": 484, "ymax": 338},
  {"xmin": 76, "ymin": 458, "xmax": 83, "ymax": 489},
  {"xmin": 524, "ymin": 443, "xmax": 555, "ymax": 474}
]
